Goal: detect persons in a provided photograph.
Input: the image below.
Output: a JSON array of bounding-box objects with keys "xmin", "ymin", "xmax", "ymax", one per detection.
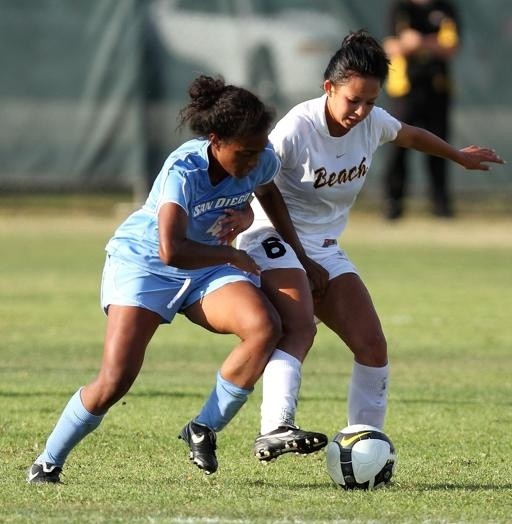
[
  {"xmin": 383, "ymin": 0, "xmax": 462, "ymax": 219},
  {"xmin": 28, "ymin": 75, "xmax": 329, "ymax": 481},
  {"xmin": 215, "ymin": 30, "xmax": 507, "ymax": 461}
]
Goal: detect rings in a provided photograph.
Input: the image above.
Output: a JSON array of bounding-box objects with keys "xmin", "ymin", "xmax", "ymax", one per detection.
[{"xmin": 230, "ymin": 228, "xmax": 235, "ymax": 231}]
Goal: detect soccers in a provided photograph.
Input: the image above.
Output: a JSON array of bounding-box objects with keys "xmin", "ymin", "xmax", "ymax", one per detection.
[{"xmin": 325, "ymin": 424, "xmax": 394, "ymax": 489}]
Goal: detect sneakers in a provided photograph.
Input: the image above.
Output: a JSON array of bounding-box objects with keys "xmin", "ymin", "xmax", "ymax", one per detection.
[
  {"xmin": 28, "ymin": 454, "xmax": 63, "ymax": 484},
  {"xmin": 177, "ymin": 415, "xmax": 219, "ymax": 476},
  {"xmin": 253, "ymin": 425, "xmax": 329, "ymax": 462}
]
